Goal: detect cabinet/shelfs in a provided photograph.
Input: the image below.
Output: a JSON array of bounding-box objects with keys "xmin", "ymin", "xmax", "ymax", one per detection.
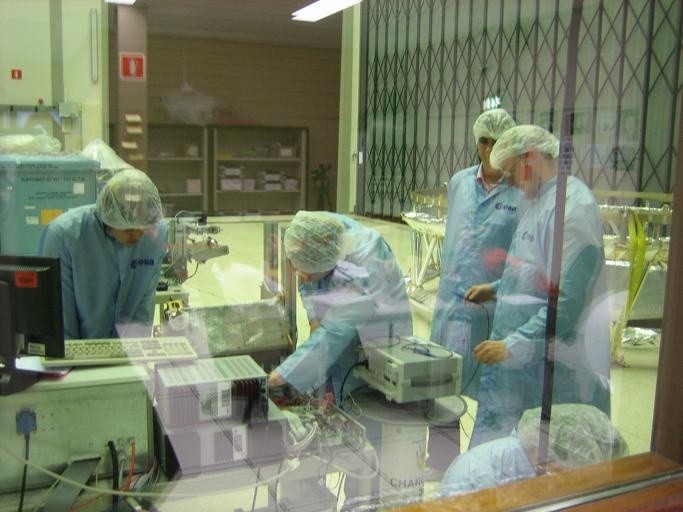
[{"xmin": 146, "ymin": 117, "xmax": 310, "ymax": 218}]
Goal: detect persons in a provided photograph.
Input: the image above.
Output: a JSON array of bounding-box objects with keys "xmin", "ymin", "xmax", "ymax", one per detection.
[
  {"xmin": 38, "ymin": 169, "xmax": 168, "ymax": 339},
  {"xmin": 262, "ymin": 211, "xmax": 412, "ymax": 389}
]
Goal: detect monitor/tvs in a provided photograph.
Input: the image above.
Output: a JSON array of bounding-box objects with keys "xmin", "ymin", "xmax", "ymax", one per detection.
[{"xmin": 0, "ymin": 256, "xmax": 64, "ymax": 396}]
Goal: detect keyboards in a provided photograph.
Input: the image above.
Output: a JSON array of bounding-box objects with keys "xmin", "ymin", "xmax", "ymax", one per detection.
[{"xmin": 41, "ymin": 336, "xmax": 197, "ymax": 368}]
